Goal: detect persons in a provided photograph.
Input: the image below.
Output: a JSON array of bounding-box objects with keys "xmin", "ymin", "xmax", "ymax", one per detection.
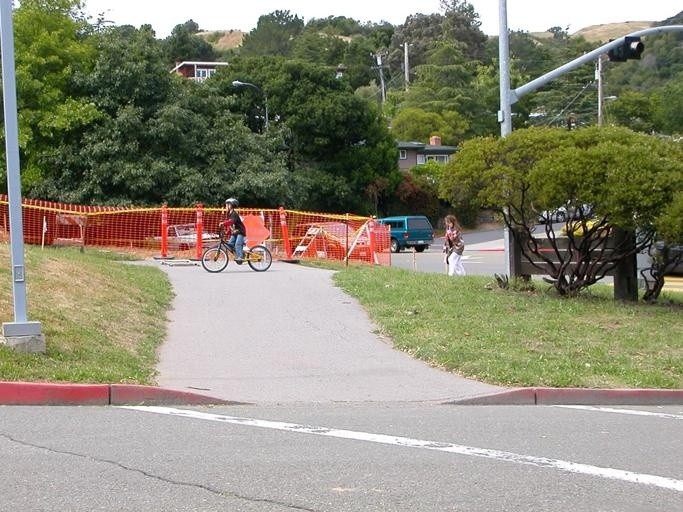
[
  {"xmin": 218, "ymin": 198, "xmax": 246, "ymax": 262},
  {"xmin": 443, "ymin": 215, "xmax": 466, "ymax": 277}
]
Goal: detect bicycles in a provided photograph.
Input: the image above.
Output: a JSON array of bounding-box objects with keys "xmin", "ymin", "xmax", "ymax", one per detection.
[{"xmin": 201, "ymin": 224, "xmax": 272, "ymax": 273}]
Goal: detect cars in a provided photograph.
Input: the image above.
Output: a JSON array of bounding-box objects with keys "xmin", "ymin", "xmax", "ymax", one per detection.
[
  {"xmin": 536, "ymin": 201, "xmax": 611, "ymax": 240},
  {"xmin": 289, "ymin": 216, "xmax": 434, "ymax": 260},
  {"xmin": 652, "ymin": 242, "xmax": 683, "ymax": 272}
]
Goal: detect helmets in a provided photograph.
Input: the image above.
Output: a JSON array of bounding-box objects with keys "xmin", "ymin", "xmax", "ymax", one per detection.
[{"xmin": 225, "ymin": 198, "xmax": 240, "ymax": 209}]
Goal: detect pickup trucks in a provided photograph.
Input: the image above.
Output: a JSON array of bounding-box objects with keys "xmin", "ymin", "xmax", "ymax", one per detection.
[{"xmin": 144, "ymin": 222, "xmax": 219, "ymax": 251}]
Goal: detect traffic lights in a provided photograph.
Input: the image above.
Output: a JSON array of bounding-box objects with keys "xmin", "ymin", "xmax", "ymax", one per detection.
[{"xmin": 624, "ymin": 36, "xmax": 644, "ymax": 59}]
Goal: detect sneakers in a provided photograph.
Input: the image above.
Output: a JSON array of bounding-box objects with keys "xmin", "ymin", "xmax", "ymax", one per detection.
[{"xmin": 234, "ymin": 256, "xmax": 245, "ymax": 261}]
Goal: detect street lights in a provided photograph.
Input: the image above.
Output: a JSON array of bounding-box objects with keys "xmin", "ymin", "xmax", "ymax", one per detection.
[
  {"xmin": 232, "ymin": 81, "xmax": 269, "ymax": 131},
  {"xmin": 604, "ymin": 95, "xmax": 617, "ymax": 102}
]
